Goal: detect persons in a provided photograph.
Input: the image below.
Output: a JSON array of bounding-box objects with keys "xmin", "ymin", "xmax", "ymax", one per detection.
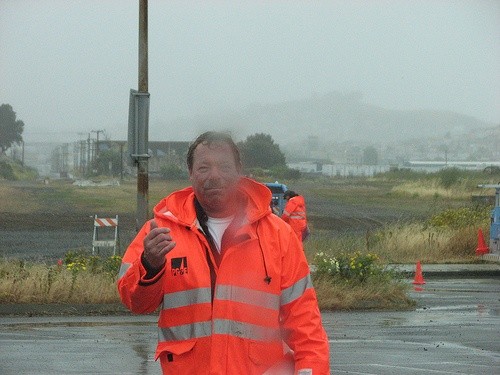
[
  {"xmin": 281, "ymin": 190, "xmax": 306, "ymax": 243},
  {"xmin": 116, "ymin": 129, "xmax": 330, "ymax": 375}
]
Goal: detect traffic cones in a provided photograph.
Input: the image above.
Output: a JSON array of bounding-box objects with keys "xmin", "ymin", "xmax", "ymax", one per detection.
[
  {"xmin": 476, "ymin": 229, "xmax": 489, "ymax": 254},
  {"xmin": 411, "ymin": 260, "xmax": 426, "ymax": 284}
]
